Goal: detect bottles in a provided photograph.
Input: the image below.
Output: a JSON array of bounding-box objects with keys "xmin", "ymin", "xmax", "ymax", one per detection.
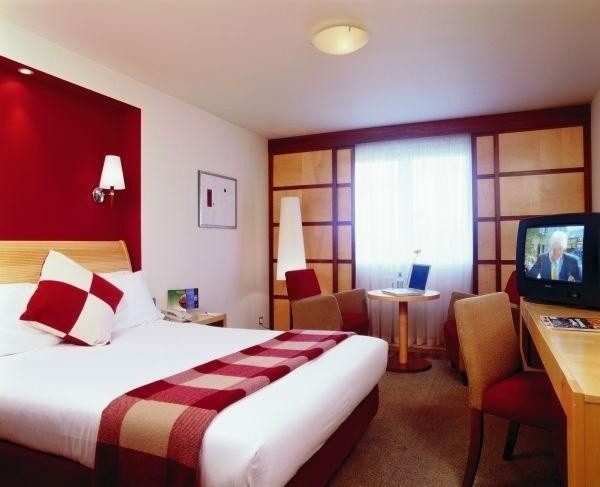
[{"xmin": 396, "ymin": 272, "xmax": 404, "ymax": 287}]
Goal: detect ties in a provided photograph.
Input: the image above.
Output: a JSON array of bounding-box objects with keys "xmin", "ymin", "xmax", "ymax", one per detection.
[{"xmin": 551, "ymin": 262, "xmax": 557, "ymax": 280}]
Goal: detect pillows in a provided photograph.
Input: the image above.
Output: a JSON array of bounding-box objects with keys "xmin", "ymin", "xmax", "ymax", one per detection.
[
  {"xmin": 0, "ymin": 283, "xmax": 64, "ymax": 357},
  {"xmin": 94, "ymin": 269, "xmax": 165, "ymax": 334},
  {"xmin": 18, "ymin": 250, "xmax": 124, "ymax": 347}
]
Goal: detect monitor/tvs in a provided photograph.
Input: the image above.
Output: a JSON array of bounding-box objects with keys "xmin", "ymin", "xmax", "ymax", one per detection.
[{"xmin": 516, "ymin": 212, "xmax": 600, "ymax": 311}]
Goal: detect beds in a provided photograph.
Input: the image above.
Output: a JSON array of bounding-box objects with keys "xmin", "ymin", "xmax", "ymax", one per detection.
[{"xmin": 0, "ymin": 240, "xmax": 389, "ymax": 487}]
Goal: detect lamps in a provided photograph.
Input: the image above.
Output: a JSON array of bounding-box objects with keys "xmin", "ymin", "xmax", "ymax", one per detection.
[
  {"xmin": 94, "ymin": 153, "xmax": 125, "ymax": 204},
  {"xmin": 276, "ymin": 197, "xmax": 307, "ymax": 331},
  {"xmin": 312, "ymin": 23, "xmax": 369, "ymax": 57},
  {"xmin": 404, "ymin": 248, "xmax": 421, "ymax": 287}
]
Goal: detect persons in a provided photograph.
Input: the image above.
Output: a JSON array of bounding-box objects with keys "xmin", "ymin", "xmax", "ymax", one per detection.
[{"xmin": 523, "ymin": 226, "xmax": 584, "ymax": 282}]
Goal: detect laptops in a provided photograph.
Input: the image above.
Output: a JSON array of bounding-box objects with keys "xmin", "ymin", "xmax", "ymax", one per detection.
[{"xmin": 381, "ymin": 264, "xmax": 432, "ymax": 296}]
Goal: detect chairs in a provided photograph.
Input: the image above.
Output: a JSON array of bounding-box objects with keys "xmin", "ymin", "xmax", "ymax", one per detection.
[
  {"xmin": 451, "ymin": 290, "xmax": 563, "ymax": 485},
  {"xmin": 285, "ymin": 268, "xmax": 369, "ymax": 336},
  {"xmin": 445, "ymin": 270, "xmax": 527, "ymax": 382}
]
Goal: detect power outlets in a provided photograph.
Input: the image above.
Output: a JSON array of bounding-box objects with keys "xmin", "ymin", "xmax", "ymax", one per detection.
[{"xmin": 259, "ymin": 317, "xmax": 264, "ymax": 325}]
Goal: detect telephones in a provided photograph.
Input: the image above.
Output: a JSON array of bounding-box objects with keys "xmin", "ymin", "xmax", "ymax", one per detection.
[{"xmin": 161, "ymin": 308, "xmax": 193, "ymax": 323}]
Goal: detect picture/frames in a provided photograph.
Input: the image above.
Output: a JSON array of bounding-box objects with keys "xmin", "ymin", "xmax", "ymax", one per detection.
[{"xmin": 198, "ymin": 170, "xmax": 237, "ymax": 229}]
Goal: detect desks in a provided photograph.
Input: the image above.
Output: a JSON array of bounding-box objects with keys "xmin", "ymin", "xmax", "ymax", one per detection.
[
  {"xmin": 367, "ymin": 289, "xmax": 440, "ymax": 373},
  {"xmin": 517, "ymin": 294, "xmax": 599, "ymax": 485}
]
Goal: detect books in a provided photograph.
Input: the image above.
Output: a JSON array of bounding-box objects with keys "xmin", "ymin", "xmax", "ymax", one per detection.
[{"xmin": 539, "ymin": 315, "xmax": 600, "ymax": 333}]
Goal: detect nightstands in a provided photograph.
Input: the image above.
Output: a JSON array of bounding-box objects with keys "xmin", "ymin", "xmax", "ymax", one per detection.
[{"xmin": 184, "ymin": 310, "xmax": 227, "ymax": 328}]
